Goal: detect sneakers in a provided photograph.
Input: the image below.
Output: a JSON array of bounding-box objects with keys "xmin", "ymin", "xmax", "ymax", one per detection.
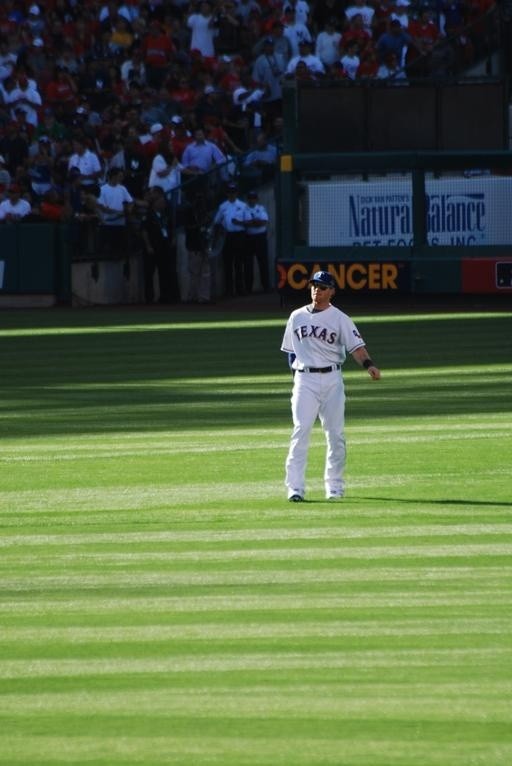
[
  {"xmin": 325, "ymin": 486, "xmax": 343, "ymax": 499},
  {"xmin": 287, "ymin": 488, "xmax": 304, "ymax": 502}
]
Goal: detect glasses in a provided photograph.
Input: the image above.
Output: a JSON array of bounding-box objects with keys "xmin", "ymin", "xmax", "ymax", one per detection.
[{"xmin": 312, "ymin": 281, "xmax": 328, "ymax": 290}]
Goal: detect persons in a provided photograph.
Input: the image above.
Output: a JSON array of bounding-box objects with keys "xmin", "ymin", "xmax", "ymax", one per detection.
[
  {"xmin": 1, "ymin": 1, "xmax": 511, "ymax": 306},
  {"xmin": 280, "ymin": 269, "xmax": 382, "ymax": 502}
]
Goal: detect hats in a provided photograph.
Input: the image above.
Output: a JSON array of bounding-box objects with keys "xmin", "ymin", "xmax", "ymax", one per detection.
[
  {"xmin": 263, "ymin": 4, "xmax": 312, "ymax": 46},
  {"xmin": 7, "ymin": 182, "xmax": 22, "ymax": 193},
  {"xmin": 248, "ymin": 191, "xmax": 257, "ymax": 197},
  {"xmin": 171, "ymin": 115, "xmax": 183, "ymax": 124},
  {"xmin": 31, "ymin": 37, "xmax": 44, "ymax": 47},
  {"xmin": 15, "ymin": 107, "xmax": 27, "ymax": 113},
  {"xmin": 150, "ymin": 122, "xmax": 163, "ymax": 134},
  {"xmin": 309, "ymin": 271, "xmax": 337, "ymax": 288},
  {"xmin": 28, "ymin": 4, "xmax": 40, "ymax": 14}
]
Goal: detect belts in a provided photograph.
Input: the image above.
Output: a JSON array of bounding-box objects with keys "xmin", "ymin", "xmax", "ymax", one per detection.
[{"xmin": 298, "ymin": 364, "xmax": 341, "ymax": 372}]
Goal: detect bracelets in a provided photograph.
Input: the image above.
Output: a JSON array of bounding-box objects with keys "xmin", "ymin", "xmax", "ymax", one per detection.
[{"xmin": 364, "ymin": 359, "xmax": 373, "ymax": 369}]
